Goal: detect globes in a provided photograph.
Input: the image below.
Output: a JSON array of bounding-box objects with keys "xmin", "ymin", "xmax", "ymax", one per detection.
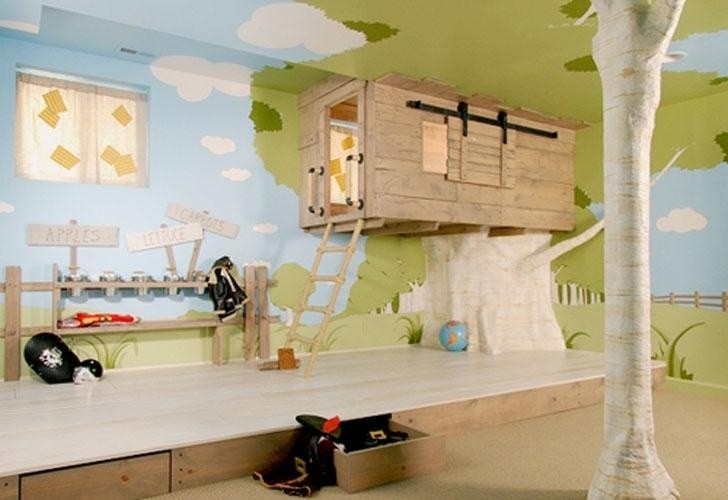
[{"xmin": 439, "ymin": 320, "xmax": 468, "ymax": 350}]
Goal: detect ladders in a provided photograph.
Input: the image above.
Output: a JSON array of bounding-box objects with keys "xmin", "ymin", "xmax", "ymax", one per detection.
[{"xmin": 283, "ymin": 219, "xmax": 364, "ymax": 378}]
